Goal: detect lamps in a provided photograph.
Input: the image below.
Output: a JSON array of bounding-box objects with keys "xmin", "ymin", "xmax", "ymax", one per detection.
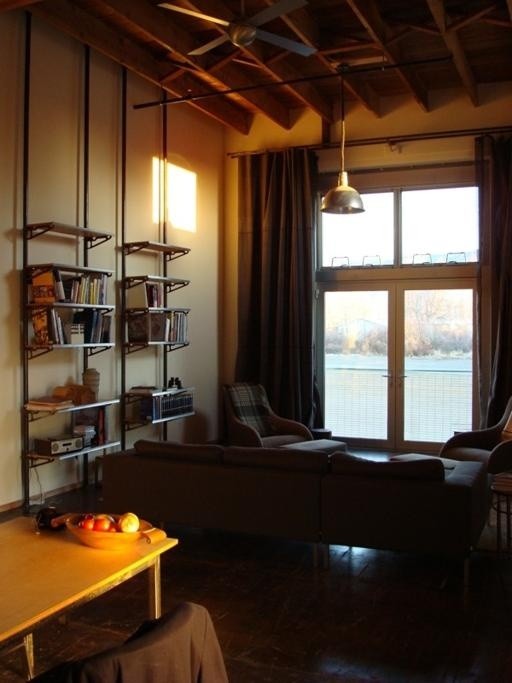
[{"xmin": 317, "ymin": 65, "xmax": 366, "ymax": 212}]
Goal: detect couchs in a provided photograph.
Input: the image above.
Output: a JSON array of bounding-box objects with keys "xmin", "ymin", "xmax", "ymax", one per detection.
[
  {"xmin": 99, "ymin": 445, "xmax": 321, "ymax": 550},
  {"xmin": 323, "ymin": 446, "xmax": 493, "ymax": 555}
]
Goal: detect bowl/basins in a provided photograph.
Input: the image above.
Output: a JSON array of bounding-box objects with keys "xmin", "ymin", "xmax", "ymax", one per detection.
[{"xmin": 66, "ymin": 514, "xmax": 155, "ymax": 548}]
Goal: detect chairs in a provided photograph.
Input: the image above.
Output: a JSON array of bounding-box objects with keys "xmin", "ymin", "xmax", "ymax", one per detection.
[
  {"xmin": 439, "ymin": 392, "xmax": 512, "ymax": 482},
  {"xmin": 24, "ymin": 603, "xmax": 233, "ymax": 682},
  {"xmin": 223, "ymin": 377, "xmax": 314, "ymax": 445}
]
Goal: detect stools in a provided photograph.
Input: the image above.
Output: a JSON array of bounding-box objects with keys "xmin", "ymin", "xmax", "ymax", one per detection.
[
  {"xmin": 278, "ymin": 438, "xmax": 346, "ymax": 454},
  {"xmin": 389, "ymin": 453, "xmax": 460, "ymax": 470},
  {"xmin": 488, "ymin": 474, "xmax": 511, "ymax": 558}
]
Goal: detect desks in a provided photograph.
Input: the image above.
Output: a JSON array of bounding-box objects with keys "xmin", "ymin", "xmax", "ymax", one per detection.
[{"xmin": 1, "ymin": 507, "xmax": 180, "ymax": 680}]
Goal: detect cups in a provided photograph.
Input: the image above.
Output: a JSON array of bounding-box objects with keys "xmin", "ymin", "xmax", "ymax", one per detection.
[{"xmin": 71, "ymin": 323, "xmax": 84, "ymax": 344}]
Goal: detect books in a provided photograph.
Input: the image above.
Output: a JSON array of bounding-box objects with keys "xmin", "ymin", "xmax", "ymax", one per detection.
[
  {"xmin": 31, "ymin": 273, "xmax": 113, "ymax": 346},
  {"xmin": 23, "ymin": 396, "xmax": 71, "ymax": 418},
  {"xmin": 141, "ymin": 392, "xmax": 193, "ymax": 421},
  {"xmin": 127, "ymin": 282, "xmax": 190, "ymax": 345}
]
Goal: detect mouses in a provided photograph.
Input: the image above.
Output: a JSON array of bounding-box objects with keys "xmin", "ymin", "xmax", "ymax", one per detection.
[{"xmin": 36, "ymin": 505, "xmax": 64, "ymax": 531}]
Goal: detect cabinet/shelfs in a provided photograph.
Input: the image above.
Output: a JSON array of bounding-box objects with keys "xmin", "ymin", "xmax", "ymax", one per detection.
[
  {"xmin": 28, "ymin": 219, "xmax": 125, "ymax": 467},
  {"xmin": 124, "ymin": 232, "xmax": 194, "ymax": 434}
]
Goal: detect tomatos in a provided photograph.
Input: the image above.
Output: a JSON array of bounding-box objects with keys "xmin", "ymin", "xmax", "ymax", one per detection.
[{"xmin": 83, "ymin": 514, "xmax": 113, "ymax": 532}]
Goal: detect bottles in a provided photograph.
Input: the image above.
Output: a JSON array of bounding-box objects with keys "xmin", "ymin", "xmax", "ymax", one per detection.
[{"xmin": 82, "ymin": 367, "xmax": 100, "ymax": 400}]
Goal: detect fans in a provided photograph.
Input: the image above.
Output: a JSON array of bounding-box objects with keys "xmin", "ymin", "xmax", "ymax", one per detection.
[{"xmin": 145, "ymin": 3, "xmax": 329, "ymax": 58}]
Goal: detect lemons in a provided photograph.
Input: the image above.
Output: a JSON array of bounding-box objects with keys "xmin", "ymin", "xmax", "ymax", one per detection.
[{"xmin": 118, "ymin": 512, "xmax": 140, "ymax": 533}]
